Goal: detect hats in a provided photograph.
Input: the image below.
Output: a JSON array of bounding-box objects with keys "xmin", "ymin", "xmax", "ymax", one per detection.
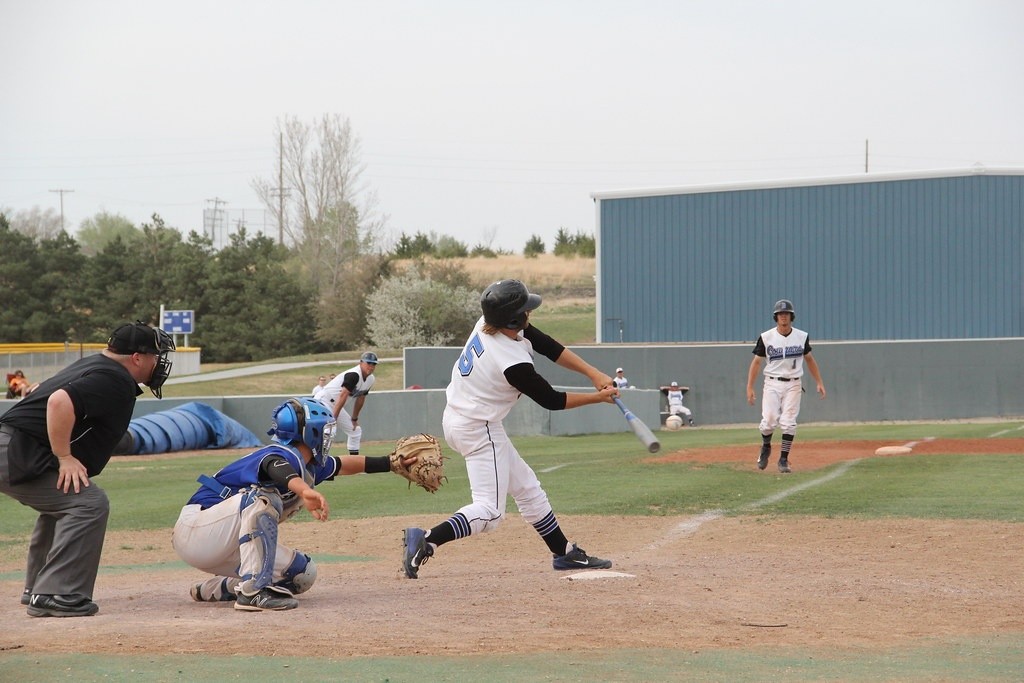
[
  {"xmin": 107, "ymin": 320, "xmax": 167, "ymax": 355},
  {"xmin": 616, "ymin": 368, "xmax": 623, "ymax": 373},
  {"xmin": 671, "ymin": 382, "xmax": 678, "ymax": 386}
]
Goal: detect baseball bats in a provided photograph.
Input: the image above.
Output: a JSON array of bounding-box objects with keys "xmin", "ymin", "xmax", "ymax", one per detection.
[{"xmin": 610, "ymin": 392, "xmax": 662, "ymax": 454}]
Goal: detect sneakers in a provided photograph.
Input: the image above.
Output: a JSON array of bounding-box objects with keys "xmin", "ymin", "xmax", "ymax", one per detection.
[
  {"xmin": 757, "ymin": 447, "xmax": 771, "ymax": 469},
  {"xmin": 401, "ymin": 528, "xmax": 438, "ymax": 579},
  {"xmin": 189, "ymin": 583, "xmax": 203, "ymax": 601},
  {"xmin": 234, "ymin": 580, "xmax": 296, "ymax": 611},
  {"xmin": 777, "ymin": 457, "xmax": 792, "ymax": 473},
  {"xmin": 21, "ymin": 587, "xmax": 98, "ymax": 617},
  {"xmin": 552, "ymin": 542, "xmax": 612, "ymax": 570}
]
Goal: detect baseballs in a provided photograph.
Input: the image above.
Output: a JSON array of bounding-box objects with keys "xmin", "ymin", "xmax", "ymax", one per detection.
[{"xmin": 666, "ymin": 415, "xmax": 683, "ymax": 430}]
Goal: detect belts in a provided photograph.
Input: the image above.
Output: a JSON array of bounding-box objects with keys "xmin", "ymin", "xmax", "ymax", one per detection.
[{"xmin": 770, "ymin": 377, "xmax": 799, "ymax": 381}]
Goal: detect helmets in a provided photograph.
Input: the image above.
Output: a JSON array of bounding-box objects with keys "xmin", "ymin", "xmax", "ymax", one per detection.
[
  {"xmin": 360, "ymin": 352, "xmax": 378, "ymax": 364},
  {"xmin": 481, "ymin": 279, "xmax": 542, "ymax": 330},
  {"xmin": 266, "ymin": 396, "xmax": 337, "ymax": 466},
  {"xmin": 772, "ymin": 299, "xmax": 795, "ymax": 323}
]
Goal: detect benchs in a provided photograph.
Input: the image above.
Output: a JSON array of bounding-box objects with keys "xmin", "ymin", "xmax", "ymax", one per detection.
[{"xmin": 660, "ymin": 412, "xmax": 680, "ymax": 414}]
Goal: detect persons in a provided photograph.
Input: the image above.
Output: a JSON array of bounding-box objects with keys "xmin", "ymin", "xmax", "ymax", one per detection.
[
  {"xmin": 313, "ymin": 351, "xmax": 378, "ymax": 455},
  {"xmin": 614, "ymin": 367, "xmax": 630, "ymax": 389},
  {"xmin": 660, "ymin": 382, "xmax": 697, "ymax": 427},
  {"xmin": 172, "ymin": 397, "xmax": 444, "ymax": 611},
  {"xmin": 9, "ymin": 370, "xmax": 39, "ymax": 400},
  {"xmin": 746, "ymin": 299, "xmax": 826, "ymax": 473},
  {"xmin": 0, "ymin": 319, "xmax": 169, "ymax": 618},
  {"xmin": 402, "ymin": 279, "xmax": 621, "ymax": 579},
  {"xmin": 312, "ymin": 374, "xmax": 337, "ymax": 394}
]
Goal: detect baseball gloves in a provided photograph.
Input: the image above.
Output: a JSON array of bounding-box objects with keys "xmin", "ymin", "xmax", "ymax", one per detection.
[{"xmin": 388, "ymin": 432, "xmax": 446, "ymax": 493}]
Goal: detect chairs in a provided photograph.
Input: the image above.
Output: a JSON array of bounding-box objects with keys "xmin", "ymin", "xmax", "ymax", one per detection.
[{"xmin": 6, "ymin": 374, "xmax": 30, "ymax": 399}]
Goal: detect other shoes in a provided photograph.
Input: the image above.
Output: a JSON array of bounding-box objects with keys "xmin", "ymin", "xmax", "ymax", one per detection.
[{"xmin": 689, "ymin": 420, "xmax": 697, "ymax": 427}]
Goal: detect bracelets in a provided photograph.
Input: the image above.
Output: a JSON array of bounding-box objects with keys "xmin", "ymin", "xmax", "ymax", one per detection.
[{"xmin": 352, "ymin": 417, "xmax": 358, "ymax": 421}]
